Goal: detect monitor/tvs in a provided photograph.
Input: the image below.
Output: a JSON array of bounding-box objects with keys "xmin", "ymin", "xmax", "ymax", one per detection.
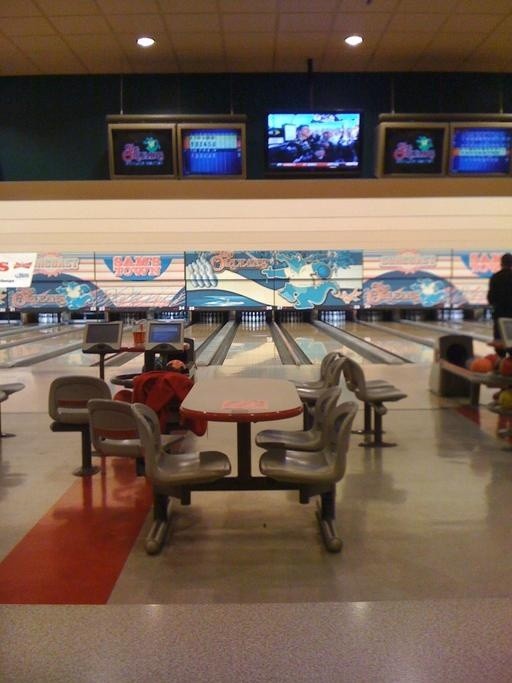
[
  {"xmin": 82, "ymin": 321, "xmax": 123, "ymax": 353},
  {"xmin": 264, "ymin": 110, "xmax": 365, "ymax": 177},
  {"xmin": 144, "ymin": 320, "xmax": 184, "ymax": 353},
  {"xmin": 498, "ymin": 317, "xmax": 512, "ymax": 352}
]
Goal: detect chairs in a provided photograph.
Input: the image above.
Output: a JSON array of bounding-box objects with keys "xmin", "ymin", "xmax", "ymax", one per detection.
[
  {"xmin": 293, "ymin": 349, "xmax": 340, "ymax": 427},
  {"xmin": 135, "ymin": 403, "xmax": 231, "ymax": 552},
  {"xmin": 87, "ymin": 397, "xmax": 143, "ymax": 487},
  {"xmin": 340, "ymin": 351, "xmax": 406, "ymax": 451},
  {"xmin": 50, "ymin": 374, "xmax": 112, "ymax": 476},
  {"xmin": 254, "ymin": 386, "xmax": 359, "ymax": 551}
]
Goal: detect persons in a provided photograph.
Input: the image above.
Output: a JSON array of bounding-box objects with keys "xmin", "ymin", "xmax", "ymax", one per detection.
[
  {"xmin": 285, "ymin": 123, "xmax": 358, "ymax": 163},
  {"xmin": 486, "ymin": 252, "xmax": 512, "ymax": 356}
]
